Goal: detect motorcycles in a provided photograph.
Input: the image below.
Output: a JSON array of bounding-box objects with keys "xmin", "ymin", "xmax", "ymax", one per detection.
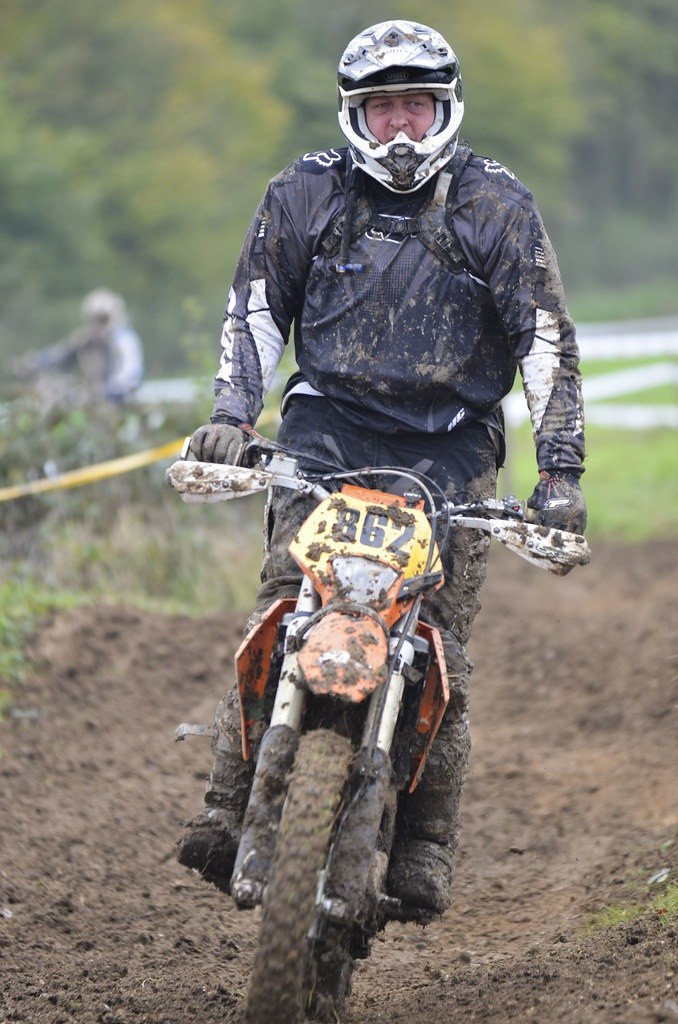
[{"xmin": 165, "ymin": 431, "xmax": 588, "ymax": 1023}]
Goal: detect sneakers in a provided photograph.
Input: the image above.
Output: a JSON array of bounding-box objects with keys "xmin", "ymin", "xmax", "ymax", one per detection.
[
  {"xmin": 386, "ymin": 833, "xmax": 454, "ymax": 914},
  {"xmin": 174, "ymin": 802, "xmax": 237, "ymax": 898}
]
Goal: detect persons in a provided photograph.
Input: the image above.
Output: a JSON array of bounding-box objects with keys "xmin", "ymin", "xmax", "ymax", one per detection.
[
  {"xmin": 179, "ymin": 19, "xmax": 588, "ymax": 913},
  {"xmin": 14, "ymin": 287, "xmax": 144, "ymax": 403}
]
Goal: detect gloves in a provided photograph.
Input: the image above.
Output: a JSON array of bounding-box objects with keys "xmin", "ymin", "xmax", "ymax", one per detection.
[
  {"xmin": 190, "ymin": 418, "xmax": 247, "ymax": 465},
  {"xmin": 528, "ymin": 472, "xmax": 588, "ymax": 536}
]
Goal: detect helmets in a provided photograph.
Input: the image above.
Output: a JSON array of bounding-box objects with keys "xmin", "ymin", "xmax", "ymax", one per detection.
[{"xmin": 337, "ymin": 20, "xmax": 464, "ymax": 193}]
